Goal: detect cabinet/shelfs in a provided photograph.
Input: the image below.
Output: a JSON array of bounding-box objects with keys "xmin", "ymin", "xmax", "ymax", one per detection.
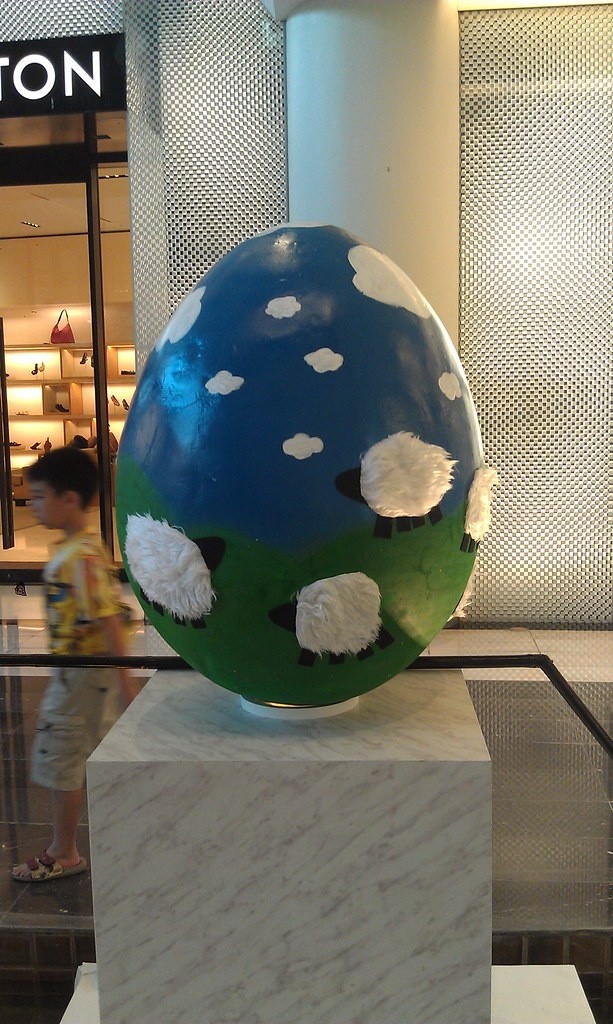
[{"xmin": 1, "ymin": 345, "xmax": 135, "ymax": 476}]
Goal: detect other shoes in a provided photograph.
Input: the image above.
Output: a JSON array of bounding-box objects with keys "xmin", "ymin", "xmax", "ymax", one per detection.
[
  {"xmin": 91, "ymin": 355, "xmax": 95, "ymax": 367},
  {"xmin": 10, "ymin": 442, "xmax": 21, "ymax": 446},
  {"xmin": 122, "ymin": 399, "xmax": 129, "ymax": 410},
  {"xmin": 111, "ymin": 395, "xmax": 120, "ymax": 406},
  {"xmin": 30, "ymin": 441, "xmax": 41, "ymax": 449},
  {"xmin": 39, "ymin": 362, "xmax": 45, "ymax": 372},
  {"xmin": 55, "ymin": 404, "xmax": 70, "ymax": 412},
  {"xmin": 80, "ymin": 352, "xmax": 88, "ymax": 364},
  {"xmin": 121, "ymin": 370, "xmax": 135, "ymax": 375},
  {"xmin": 15, "ymin": 409, "xmax": 29, "ymax": 415},
  {"xmin": 32, "ymin": 364, "xmax": 38, "ymax": 375}
]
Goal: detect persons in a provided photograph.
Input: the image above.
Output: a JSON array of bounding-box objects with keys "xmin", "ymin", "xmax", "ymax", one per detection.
[
  {"xmin": 95, "ymin": 424, "xmax": 118, "ymax": 464},
  {"xmin": 71, "ymin": 436, "xmax": 97, "ymax": 447},
  {"xmin": 13, "ymin": 447, "xmax": 137, "ymax": 882}
]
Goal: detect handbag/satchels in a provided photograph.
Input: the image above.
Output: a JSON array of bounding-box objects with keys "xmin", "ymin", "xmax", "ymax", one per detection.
[{"xmin": 51, "ymin": 309, "xmax": 75, "ymax": 343}]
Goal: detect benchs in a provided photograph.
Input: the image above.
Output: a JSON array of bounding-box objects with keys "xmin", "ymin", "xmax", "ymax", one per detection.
[{"xmin": 14, "ymin": 466, "xmax": 32, "ymax": 507}]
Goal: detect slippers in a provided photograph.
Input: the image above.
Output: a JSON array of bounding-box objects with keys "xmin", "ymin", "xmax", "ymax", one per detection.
[{"xmin": 12, "ymin": 849, "xmax": 87, "ymax": 882}]
